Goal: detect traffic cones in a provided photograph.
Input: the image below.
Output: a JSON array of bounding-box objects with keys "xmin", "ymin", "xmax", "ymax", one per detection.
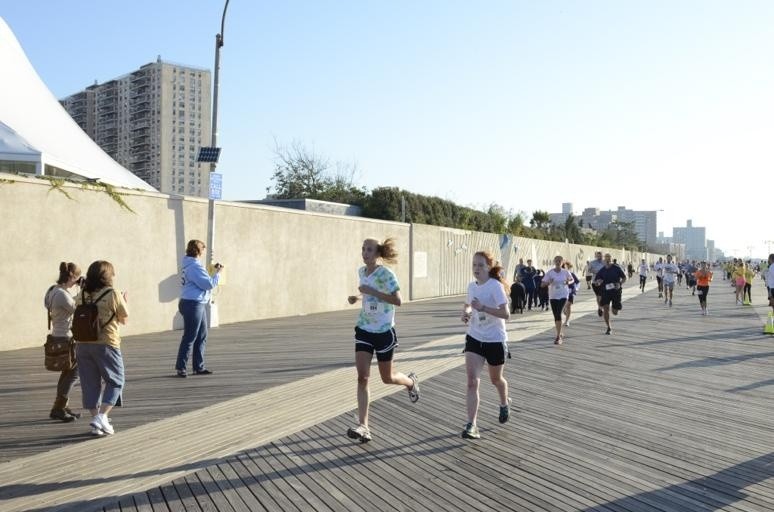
[
  {"xmin": 763, "ymin": 311, "xmax": 774, "ymax": 333},
  {"xmin": 743, "ymin": 292, "xmax": 751, "ymax": 304}
]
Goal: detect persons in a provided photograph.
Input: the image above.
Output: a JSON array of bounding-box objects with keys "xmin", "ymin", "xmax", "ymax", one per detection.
[
  {"xmin": 347, "ymin": 238, "xmax": 420, "ymax": 444},
  {"xmin": 521, "ymin": 266, "xmax": 536, "ymax": 311},
  {"xmin": 694, "ymin": 262, "xmax": 712, "ymax": 316},
  {"xmin": 462, "ymin": 252, "xmax": 512, "ymax": 439},
  {"xmin": 534, "ymin": 270, "xmax": 543, "ymax": 307},
  {"xmin": 763, "ymin": 261, "xmax": 768, "ymax": 269},
  {"xmin": 738, "ymin": 259, "xmax": 742, "ymax": 262},
  {"xmin": 75, "ymin": 261, "xmax": 129, "ymax": 436},
  {"xmin": 527, "ymin": 260, "xmax": 532, "ymax": 266},
  {"xmin": 679, "ymin": 259, "xmax": 691, "ymax": 285},
  {"xmin": 745, "ymin": 263, "xmax": 758, "ymax": 305},
  {"xmin": 636, "ymin": 259, "xmax": 650, "ymax": 293},
  {"xmin": 723, "ymin": 260, "xmax": 732, "ymax": 280},
  {"xmin": 539, "ymin": 277, "xmax": 549, "ymax": 311},
  {"xmin": 583, "ymin": 260, "xmax": 593, "ymax": 290},
  {"xmin": 621, "ymin": 259, "xmax": 635, "ymax": 279},
  {"xmin": 175, "ymin": 240, "xmax": 222, "ymax": 378},
  {"xmin": 513, "ymin": 258, "xmax": 526, "ymax": 282},
  {"xmin": 562, "ymin": 261, "xmax": 580, "ymax": 326},
  {"xmin": 768, "ymin": 254, "xmax": 774, "ymax": 314},
  {"xmin": 662, "ymin": 255, "xmax": 679, "ymax": 307},
  {"xmin": 594, "ymin": 254, "xmax": 627, "ymax": 334},
  {"xmin": 44, "ymin": 262, "xmax": 86, "ymax": 422},
  {"xmin": 759, "ymin": 260, "xmax": 763, "ymax": 269},
  {"xmin": 589, "ymin": 252, "xmax": 607, "ymax": 317},
  {"xmin": 689, "ymin": 260, "xmax": 697, "ymax": 295},
  {"xmin": 541, "ymin": 256, "xmax": 575, "ymax": 345},
  {"xmin": 735, "ymin": 263, "xmax": 745, "ymax": 305},
  {"xmin": 654, "ymin": 258, "xmax": 664, "ymax": 299},
  {"xmin": 734, "ymin": 258, "xmax": 738, "ymax": 263}
]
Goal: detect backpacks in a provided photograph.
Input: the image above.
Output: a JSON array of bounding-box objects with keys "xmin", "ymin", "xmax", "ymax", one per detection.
[{"xmin": 71, "ymin": 288, "xmax": 117, "ymax": 342}]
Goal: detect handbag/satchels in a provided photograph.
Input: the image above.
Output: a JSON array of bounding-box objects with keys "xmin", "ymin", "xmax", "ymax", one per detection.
[{"xmin": 44, "ymin": 285, "xmax": 77, "ymax": 371}]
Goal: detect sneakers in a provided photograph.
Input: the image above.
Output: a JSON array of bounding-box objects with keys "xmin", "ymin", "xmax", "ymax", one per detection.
[
  {"xmin": 66, "ymin": 407, "xmax": 82, "ymax": 418},
  {"xmin": 193, "ymin": 369, "xmax": 213, "ymax": 375},
  {"xmin": 49, "ymin": 408, "xmax": 76, "ymax": 422},
  {"xmin": 462, "ymin": 423, "xmax": 480, "ymax": 439},
  {"xmin": 606, "ymin": 329, "xmax": 612, "ymax": 335},
  {"xmin": 499, "ymin": 398, "xmax": 512, "ymax": 424},
  {"xmin": 90, "ymin": 415, "xmax": 114, "ymax": 435},
  {"xmin": 347, "ymin": 425, "xmax": 372, "ymax": 443},
  {"xmin": 564, "ymin": 322, "xmax": 569, "ymax": 327},
  {"xmin": 408, "ymin": 373, "xmax": 420, "ymax": 404},
  {"xmin": 177, "ymin": 370, "xmax": 187, "ymax": 377},
  {"xmin": 554, "ymin": 338, "xmax": 562, "ymax": 344},
  {"xmin": 89, "ymin": 417, "xmax": 111, "ymax": 436}
]
